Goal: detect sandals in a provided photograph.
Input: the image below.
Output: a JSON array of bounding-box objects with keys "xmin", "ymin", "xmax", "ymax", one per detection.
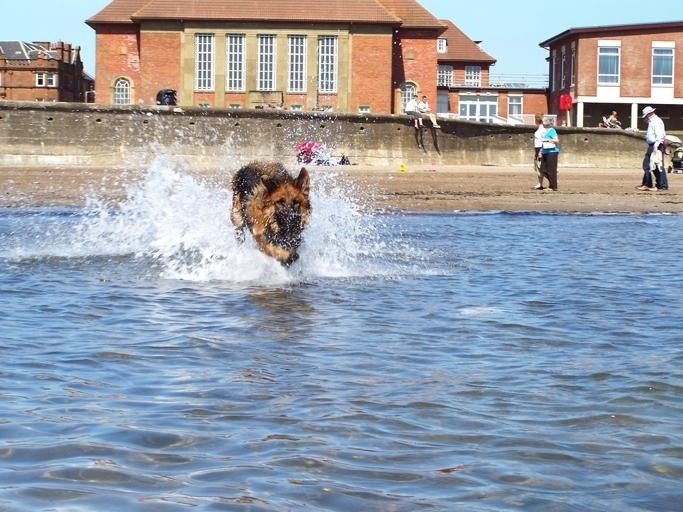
[{"xmin": 543, "ymin": 188, "xmax": 554, "ymax": 191}]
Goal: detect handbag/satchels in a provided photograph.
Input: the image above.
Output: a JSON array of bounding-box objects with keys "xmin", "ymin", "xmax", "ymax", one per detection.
[
  {"xmin": 534, "ymin": 157, "xmax": 544, "ymax": 178},
  {"xmin": 543, "ymin": 142, "xmax": 556, "ymax": 149}
]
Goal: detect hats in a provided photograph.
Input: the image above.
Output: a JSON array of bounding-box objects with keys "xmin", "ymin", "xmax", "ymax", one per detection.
[{"xmin": 641, "ymin": 106, "xmax": 657, "ymax": 119}]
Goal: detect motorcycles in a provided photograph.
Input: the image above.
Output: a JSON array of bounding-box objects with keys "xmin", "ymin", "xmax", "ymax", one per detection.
[{"xmin": 598, "ymin": 117, "xmax": 620, "ymax": 129}]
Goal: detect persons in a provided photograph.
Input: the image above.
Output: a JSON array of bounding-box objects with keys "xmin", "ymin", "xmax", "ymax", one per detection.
[
  {"xmin": 635, "ymin": 106, "xmax": 670, "ymax": 191},
  {"xmin": 418, "ymin": 95, "xmax": 440, "ymax": 129},
  {"xmin": 533, "ymin": 114, "xmax": 548, "ymax": 190},
  {"xmin": 606, "ymin": 110, "xmax": 622, "ymax": 130},
  {"xmin": 661, "ymin": 135, "xmax": 682, "ymax": 156},
  {"xmin": 539, "ymin": 117, "xmax": 559, "ymax": 192},
  {"xmin": 405, "ymin": 95, "xmax": 425, "ymax": 130}
]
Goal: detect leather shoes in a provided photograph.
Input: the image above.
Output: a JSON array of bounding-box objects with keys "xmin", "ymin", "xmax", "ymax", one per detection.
[
  {"xmin": 647, "ymin": 186, "xmax": 663, "ymax": 191},
  {"xmin": 635, "ymin": 184, "xmax": 649, "ymax": 190}
]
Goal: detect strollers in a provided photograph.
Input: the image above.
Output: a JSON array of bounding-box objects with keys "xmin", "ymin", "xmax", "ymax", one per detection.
[{"xmin": 667, "ymin": 147, "xmax": 682, "ymax": 174}]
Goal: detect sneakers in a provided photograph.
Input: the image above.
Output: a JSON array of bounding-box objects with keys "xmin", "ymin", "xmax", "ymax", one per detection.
[
  {"xmin": 415, "ymin": 125, "xmax": 442, "ymax": 130},
  {"xmin": 534, "ymin": 185, "xmax": 542, "ymax": 189}
]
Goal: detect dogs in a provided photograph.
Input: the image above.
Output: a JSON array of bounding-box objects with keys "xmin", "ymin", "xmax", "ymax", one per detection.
[{"xmin": 229, "ymin": 159, "xmax": 313, "ymax": 270}]
